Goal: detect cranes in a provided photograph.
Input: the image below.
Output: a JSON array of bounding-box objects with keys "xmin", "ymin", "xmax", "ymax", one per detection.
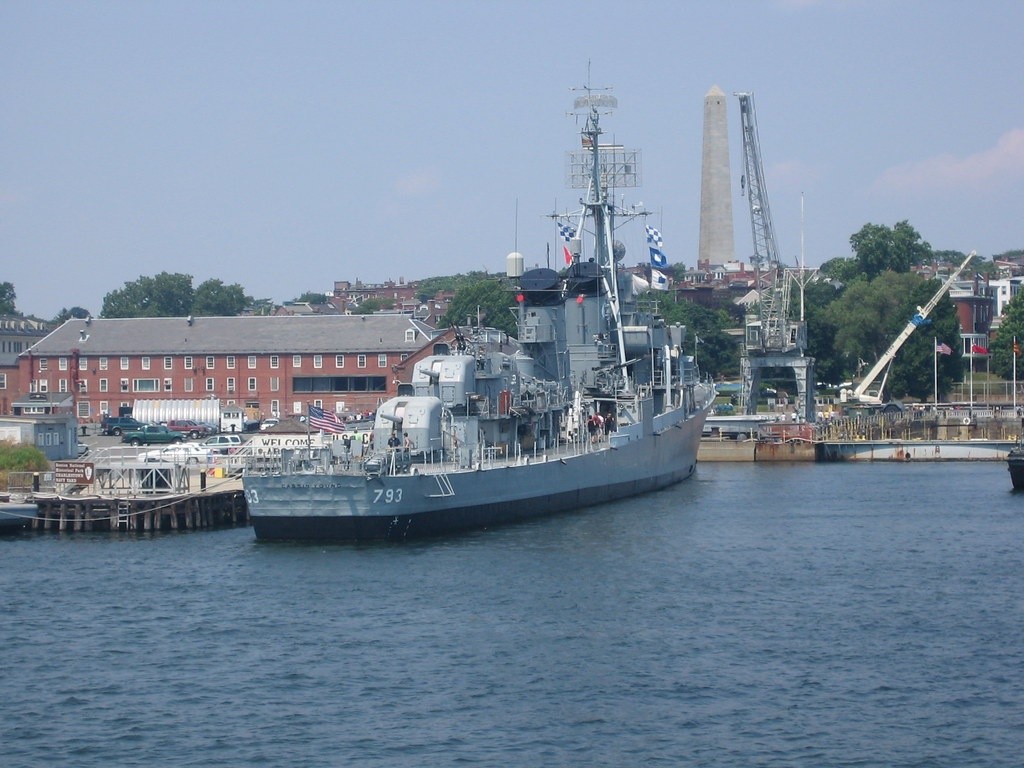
[{"xmin": 734, "ymin": 91, "xmax": 817, "ymax": 423}]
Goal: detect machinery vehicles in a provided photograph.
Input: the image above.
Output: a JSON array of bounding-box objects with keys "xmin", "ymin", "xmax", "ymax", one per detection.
[{"xmin": 838, "ymin": 249, "xmax": 977, "ymax": 414}]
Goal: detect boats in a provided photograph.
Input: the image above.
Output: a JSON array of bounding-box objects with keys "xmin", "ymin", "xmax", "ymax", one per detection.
[
  {"xmin": 1004, "ymin": 418, "xmax": 1024, "ymax": 492},
  {"xmin": 235, "ymin": 59, "xmax": 718, "ymax": 543}
]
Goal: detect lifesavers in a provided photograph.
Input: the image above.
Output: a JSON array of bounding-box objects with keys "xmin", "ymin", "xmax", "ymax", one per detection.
[{"xmin": 963, "ymin": 417, "xmax": 970, "ymax": 425}]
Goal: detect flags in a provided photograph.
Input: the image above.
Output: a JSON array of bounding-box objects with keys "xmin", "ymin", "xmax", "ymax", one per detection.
[
  {"xmin": 1013, "ymin": 341, "xmax": 1022, "ymax": 358},
  {"xmin": 645, "ymin": 225, "xmax": 663, "ymax": 247},
  {"xmin": 308, "ymin": 405, "xmax": 345, "ymax": 434},
  {"xmin": 563, "ymin": 245, "xmax": 573, "ymax": 264},
  {"xmin": 937, "ymin": 339, "xmax": 954, "ymax": 356},
  {"xmin": 650, "ymin": 247, "xmax": 667, "ymax": 266},
  {"xmin": 557, "ymin": 222, "xmax": 576, "ymax": 242},
  {"xmin": 651, "ymin": 268, "xmax": 668, "ymax": 291},
  {"xmin": 972, "ymin": 343, "xmax": 990, "ymax": 356}
]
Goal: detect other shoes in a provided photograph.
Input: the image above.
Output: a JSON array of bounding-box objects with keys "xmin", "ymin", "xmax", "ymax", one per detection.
[{"xmin": 592, "ymin": 441, "xmax": 597, "ymax": 444}]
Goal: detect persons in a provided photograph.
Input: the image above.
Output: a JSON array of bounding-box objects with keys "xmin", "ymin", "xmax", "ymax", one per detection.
[
  {"xmin": 587, "ymin": 412, "xmax": 617, "ymax": 444},
  {"xmin": 387, "ymin": 432, "xmax": 412, "ymax": 469},
  {"xmin": 781, "ymin": 407, "xmax": 845, "ymax": 422},
  {"xmin": 342, "ymin": 409, "xmax": 373, "ymax": 422}
]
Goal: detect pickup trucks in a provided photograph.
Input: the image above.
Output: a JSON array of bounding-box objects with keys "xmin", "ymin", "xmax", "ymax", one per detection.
[
  {"xmin": 99, "ymin": 417, "xmax": 149, "ymax": 438},
  {"xmin": 159, "ymin": 419, "xmax": 219, "ymax": 439},
  {"xmin": 121, "ymin": 424, "xmax": 187, "ymax": 448}
]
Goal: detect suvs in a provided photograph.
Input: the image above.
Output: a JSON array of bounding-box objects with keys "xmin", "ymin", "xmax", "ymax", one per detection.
[{"xmin": 200, "ymin": 435, "xmax": 242, "ymax": 453}]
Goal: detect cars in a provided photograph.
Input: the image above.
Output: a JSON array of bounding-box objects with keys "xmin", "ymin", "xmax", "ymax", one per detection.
[
  {"xmin": 260, "ymin": 418, "xmax": 280, "ymax": 432},
  {"xmin": 137, "ymin": 442, "xmax": 213, "ymax": 466}
]
